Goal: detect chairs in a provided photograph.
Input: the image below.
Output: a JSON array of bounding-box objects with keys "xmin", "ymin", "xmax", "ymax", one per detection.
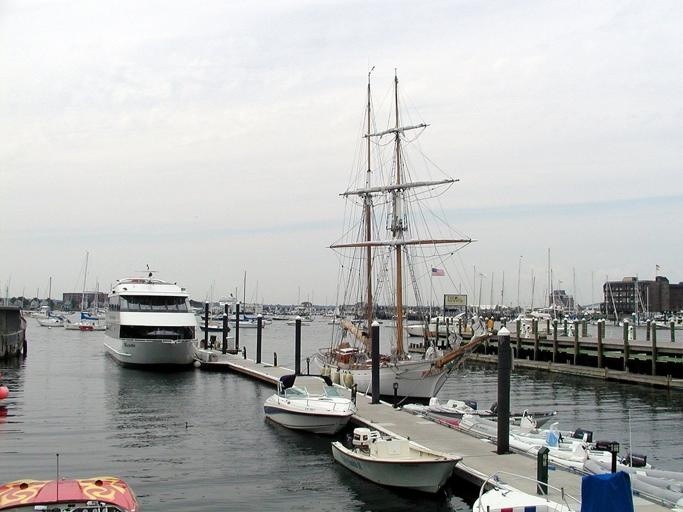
[{"xmin": 351, "ymin": 427, "xmax": 381, "ymax": 454}]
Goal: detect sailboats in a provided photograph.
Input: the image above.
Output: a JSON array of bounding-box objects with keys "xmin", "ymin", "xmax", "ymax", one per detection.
[
  {"xmin": 0, "ymin": 250, "xmax": 103, "ymax": 364},
  {"xmin": 310, "ymin": 67, "xmax": 493, "ymax": 407},
  {"xmin": 200, "ymin": 245, "xmax": 683, "ymax": 340}
]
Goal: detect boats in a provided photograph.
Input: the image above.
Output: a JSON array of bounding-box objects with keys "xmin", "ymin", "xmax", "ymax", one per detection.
[
  {"xmin": 0, "ymin": 454, "xmax": 139, "ymax": 512},
  {"xmin": 330, "ymin": 426, "xmax": 460, "ymax": 492},
  {"xmin": 263, "ymin": 373, "xmax": 355, "ymax": 434},
  {"xmin": 403, "ymin": 396, "xmax": 683, "ymax": 512},
  {"xmin": 102, "ymin": 264, "xmax": 198, "ymax": 369}
]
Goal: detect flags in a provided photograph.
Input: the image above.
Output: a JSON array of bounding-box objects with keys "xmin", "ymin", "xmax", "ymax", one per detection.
[{"xmin": 431, "ymin": 267, "xmax": 445, "ymax": 276}]
